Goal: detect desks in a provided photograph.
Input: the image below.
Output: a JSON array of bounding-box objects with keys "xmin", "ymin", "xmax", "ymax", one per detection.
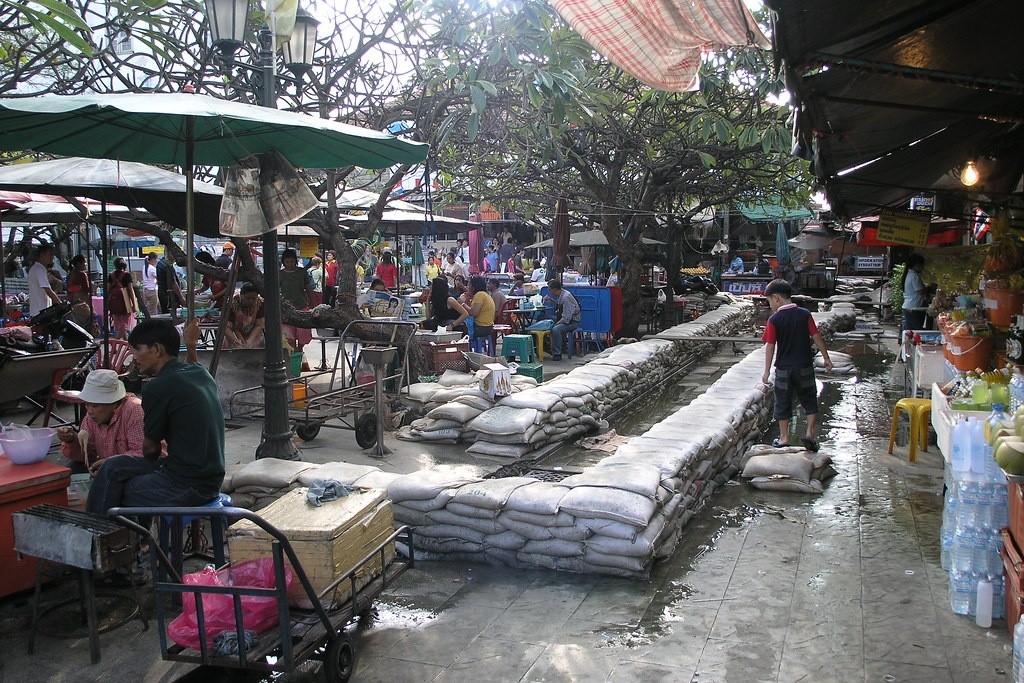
[
  {"xmin": 133, "ymin": 314, "xmax": 219, "ymax": 346},
  {"xmin": 311, "ymin": 336, "xmax": 356, "ymax": 371},
  {"xmin": 808, "ymin": 297, "xmax": 892, "ymax": 326},
  {"xmin": 503, "ymin": 307, "xmax": 556, "ymax": 355},
  {"xmin": 0, "ymin": 452, "xmax": 72, "ymax": 597}
]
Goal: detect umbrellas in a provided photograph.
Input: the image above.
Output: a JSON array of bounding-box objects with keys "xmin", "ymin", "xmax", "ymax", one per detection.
[
  {"xmin": 0, "ymin": 86, "xmax": 430, "ymax": 552},
  {"xmin": 79, "ymin": 228, "xmax": 155, "ymax": 273},
  {"xmin": 412, "ymin": 235, "xmax": 425, "ymax": 287},
  {"xmin": 776, "ymin": 219, "xmax": 791, "ymax": 273},
  {"xmin": 345, "ymin": 207, "xmax": 485, "ymax": 297},
  {"xmin": 0, "ymin": 157, "xmax": 226, "ymax": 368},
  {"xmin": 524, "ymin": 226, "xmax": 667, "ymax": 287}
]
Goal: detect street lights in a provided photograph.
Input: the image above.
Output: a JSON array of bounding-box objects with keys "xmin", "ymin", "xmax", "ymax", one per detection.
[{"xmin": 204, "ymin": 0, "xmax": 321, "ymax": 461}]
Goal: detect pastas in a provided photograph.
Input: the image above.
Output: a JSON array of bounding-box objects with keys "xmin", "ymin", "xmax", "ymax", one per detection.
[{"xmin": 78, "ymin": 430, "xmax": 89, "ymax": 469}]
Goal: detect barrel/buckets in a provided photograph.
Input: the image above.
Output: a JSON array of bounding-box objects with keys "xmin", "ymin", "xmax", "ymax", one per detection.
[
  {"xmin": 939, "ymin": 287, "xmax": 1024, "ymax": 370},
  {"xmin": 290, "ymin": 352, "xmax": 303, "ymax": 377},
  {"xmin": 293, "ymin": 383, "xmax": 309, "ymax": 410}
]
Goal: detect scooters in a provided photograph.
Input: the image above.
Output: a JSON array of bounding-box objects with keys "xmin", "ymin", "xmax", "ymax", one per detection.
[{"xmin": 0, "ymin": 298, "xmax": 99, "ymax": 393}]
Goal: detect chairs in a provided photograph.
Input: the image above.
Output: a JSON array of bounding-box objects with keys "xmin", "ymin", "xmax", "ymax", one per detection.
[{"xmin": 43, "ymin": 338, "xmax": 136, "ymax": 430}]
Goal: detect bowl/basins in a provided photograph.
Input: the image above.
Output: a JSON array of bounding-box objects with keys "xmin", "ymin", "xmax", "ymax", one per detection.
[
  {"xmin": 535, "ymin": 306, "xmax": 546, "ymax": 309},
  {"xmin": 0, "ymin": 428, "xmax": 57, "ymax": 464}
]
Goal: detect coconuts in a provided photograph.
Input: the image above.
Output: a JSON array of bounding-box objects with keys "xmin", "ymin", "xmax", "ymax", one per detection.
[{"xmin": 990, "ymin": 405, "xmax": 1024, "ymax": 476}]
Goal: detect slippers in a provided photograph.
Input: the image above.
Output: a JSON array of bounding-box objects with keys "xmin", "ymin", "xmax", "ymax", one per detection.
[
  {"xmin": 772, "ymin": 439, "xmax": 791, "ymax": 447},
  {"xmin": 93, "ymin": 570, "xmax": 148, "ymax": 587},
  {"xmin": 800, "ymin": 437, "xmax": 820, "ymax": 452}
]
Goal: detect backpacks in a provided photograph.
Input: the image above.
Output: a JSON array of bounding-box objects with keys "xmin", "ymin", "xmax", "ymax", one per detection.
[
  {"xmin": 108, "ymin": 271, "xmax": 129, "ymax": 314},
  {"xmin": 308, "ymin": 268, "xmax": 321, "ymax": 290}
]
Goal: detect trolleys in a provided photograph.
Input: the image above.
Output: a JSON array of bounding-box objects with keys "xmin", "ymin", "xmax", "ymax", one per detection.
[
  {"xmin": 229, "ymin": 320, "xmax": 420, "ymax": 448},
  {"xmin": 105, "ymin": 506, "xmax": 413, "ymax": 683}
]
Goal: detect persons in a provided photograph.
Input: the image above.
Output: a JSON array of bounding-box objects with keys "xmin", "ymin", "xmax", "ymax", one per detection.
[
  {"xmin": 897, "ymin": 254, "xmax": 938, "ymax": 362},
  {"xmin": 57, "ymin": 370, "xmax": 168, "ymax": 551},
  {"xmin": 28, "ymin": 246, "xmax": 187, "ymax": 377},
  {"xmin": 657, "ymin": 260, "xmax": 667, "ymax": 278},
  {"xmin": 761, "ymin": 279, "xmax": 833, "ymax": 453},
  {"xmin": 729, "ymin": 254, "xmax": 744, "ymax": 274},
  {"xmin": 427, "ymin": 227, "xmax": 545, "ymax": 341},
  {"xmin": 40, "ymin": 319, "xmax": 226, "ymax": 588},
  {"xmin": 543, "ymin": 278, "xmax": 582, "ymax": 361},
  {"xmin": 385, "ymin": 298, "xmax": 400, "ymax": 314},
  {"xmin": 757, "ymin": 256, "xmax": 773, "ymax": 274},
  {"xmin": 755, "ymin": 238, "xmax": 762, "ymax": 252},
  {"xmin": 356, "ymin": 246, "xmax": 378, "ymax": 283},
  {"xmin": 360, "ymin": 278, "xmax": 405, "ymax": 392},
  {"xmin": 194, "ymin": 243, "xmax": 338, "ymax": 353},
  {"xmin": 377, "ymin": 246, "xmax": 398, "ymax": 288}
]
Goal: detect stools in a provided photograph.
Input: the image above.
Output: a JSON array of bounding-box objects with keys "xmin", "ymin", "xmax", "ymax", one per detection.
[
  {"xmin": 465, "ymin": 318, "xmax": 587, "ymax": 364},
  {"xmin": 888, "ymin": 397, "xmax": 933, "ymax": 463},
  {"xmin": 159, "ymin": 491, "xmax": 236, "ymax": 583}
]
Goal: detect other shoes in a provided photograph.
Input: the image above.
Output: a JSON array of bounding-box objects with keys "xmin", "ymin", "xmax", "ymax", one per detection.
[
  {"xmin": 141, "ymin": 542, "xmax": 150, "ymax": 553},
  {"xmin": 553, "ymin": 354, "xmax": 562, "ymax": 360}
]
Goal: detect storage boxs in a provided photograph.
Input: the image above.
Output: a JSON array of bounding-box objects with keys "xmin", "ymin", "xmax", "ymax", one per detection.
[
  {"xmin": 516, "ymin": 362, "xmax": 544, "ymax": 383},
  {"xmin": 420, "ymin": 341, "xmax": 470, "ymax": 375},
  {"xmin": 899, "ymin": 328, "xmax": 996, "ymax": 460},
  {"xmin": 474, "ymin": 363, "xmax": 511, "ymax": 398},
  {"xmin": 226, "ymin": 485, "xmax": 395, "ymax": 610}
]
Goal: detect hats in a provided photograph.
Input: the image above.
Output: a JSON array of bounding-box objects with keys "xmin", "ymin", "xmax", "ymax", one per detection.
[
  {"xmin": 77, "ymin": 369, "xmax": 126, "ymax": 403},
  {"xmin": 223, "ymin": 242, "xmax": 235, "ymax": 250}
]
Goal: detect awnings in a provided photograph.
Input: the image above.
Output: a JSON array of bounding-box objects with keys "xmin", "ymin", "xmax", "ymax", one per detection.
[{"xmin": 764, "ymin": 0, "xmax": 1024, "ymax": 228}]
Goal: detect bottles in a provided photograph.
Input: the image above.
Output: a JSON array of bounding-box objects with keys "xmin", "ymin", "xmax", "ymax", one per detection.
[
  {"xmin": 96, "ymin": 286, "xmax": 100, "ymax": 297},
  {"xmin": 587, "ymin": 271, "xmax": 607, "ymax": 286},
  {"xmin": 940, "ymin": 314, "xmax": 1024, "ymax": 683},
  {"xmin": 519, "ymin": 296, "xmax": 527, "ymax": 310},
  {"xmin": 47, "ymin": 335, "xmax": 52, "ymax": 351},
  {"xmin": 137, "ymin": 280, "xmax": 143, "ymax": 289},
  {"xmin": 563, "ymin": 270, "xmax": 578, "ymax": 284}
]
